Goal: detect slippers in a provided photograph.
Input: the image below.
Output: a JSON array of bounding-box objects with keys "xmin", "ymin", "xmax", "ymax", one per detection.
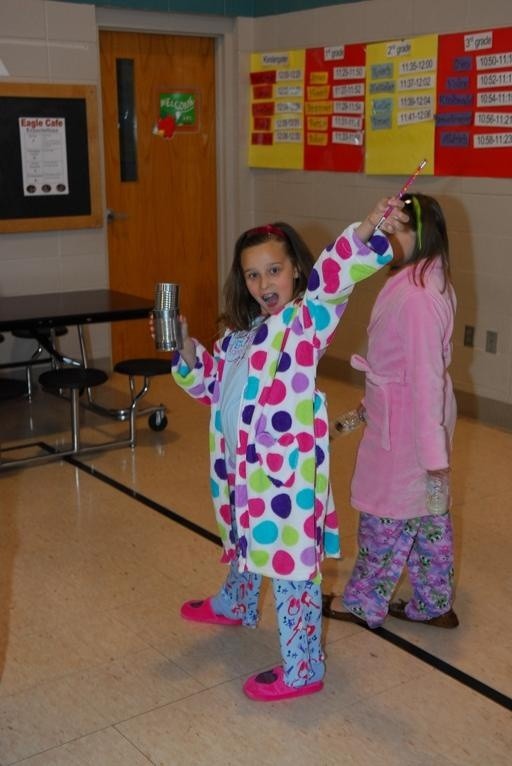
[
  {"xmin": 243, "ymin": 665, "xmax": 323, "ymax": 701},
  {"xmin": 181, "ymin": 597, "xmax": 242, "ymax": 625}
]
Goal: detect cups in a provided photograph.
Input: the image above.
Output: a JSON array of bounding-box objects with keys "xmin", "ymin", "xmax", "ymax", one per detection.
[{"xmin": 153, "ymin": 283, "xmax": 182, "ymax": 352}]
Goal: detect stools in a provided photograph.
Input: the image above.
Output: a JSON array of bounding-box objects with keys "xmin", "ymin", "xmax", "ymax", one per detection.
[{"xmin": 0, "ymin": 326, "xmax": 171, "ymax": 472}]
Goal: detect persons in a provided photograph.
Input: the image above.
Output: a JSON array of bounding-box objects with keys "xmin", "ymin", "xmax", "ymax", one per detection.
[
  {"xmin": 323, "ymin": 192, "xmax": 462, "ymax": 631},
  {"xmin": 149, "ymin": 197, "xmax": 409, "ymax": 701}
]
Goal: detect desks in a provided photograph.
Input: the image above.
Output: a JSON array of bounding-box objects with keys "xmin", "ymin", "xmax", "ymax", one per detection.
[{"xmin": 0, "ymin": 289, "xmax": 154, "ymax": 471}]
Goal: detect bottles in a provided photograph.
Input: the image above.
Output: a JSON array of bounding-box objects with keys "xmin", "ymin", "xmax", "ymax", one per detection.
[
  {"xmin": 329, "ymin": 410, "xmax": 364, "ymax": 442},
  {"xmin": 425, "ymin": 468, "xmax": 449, "ymax": 517}
]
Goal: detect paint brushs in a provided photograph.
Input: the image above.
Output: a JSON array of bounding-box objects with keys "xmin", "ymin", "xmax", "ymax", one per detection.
[{"xmin": 367, "ymin": 159, "xmax": 428, "ymax": 242}]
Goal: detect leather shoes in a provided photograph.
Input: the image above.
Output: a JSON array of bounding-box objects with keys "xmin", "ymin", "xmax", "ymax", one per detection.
[
  {"xmin": 388, "ymin": 598, "xmax": 459, "ymax": 628},
  {"xmin": 322, "ymin": 593, "xmax": 369, "ymax": 625}
]
